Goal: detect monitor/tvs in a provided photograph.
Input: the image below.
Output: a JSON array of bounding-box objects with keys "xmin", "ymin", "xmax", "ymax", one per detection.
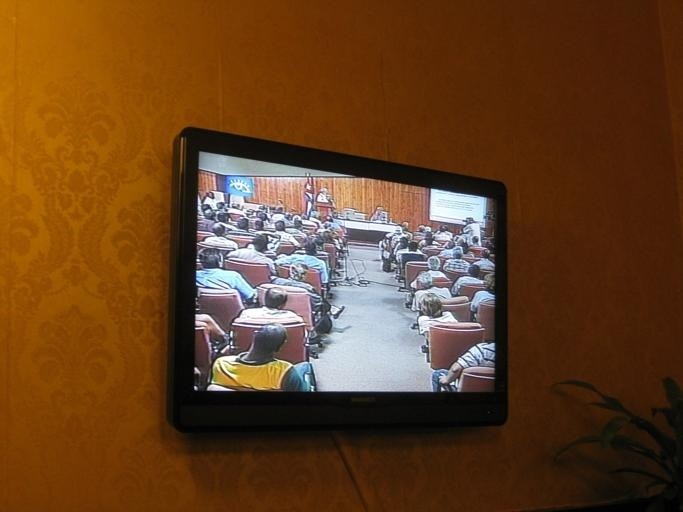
[{"xmin": 165, "ymin": 127, "xmax": 509, "ymax": 435}]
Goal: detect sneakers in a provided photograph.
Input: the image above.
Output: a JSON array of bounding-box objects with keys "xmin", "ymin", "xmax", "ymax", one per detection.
[{"xmin": 333, "ymin": 305, "xmax": 345, "ymax": 319}]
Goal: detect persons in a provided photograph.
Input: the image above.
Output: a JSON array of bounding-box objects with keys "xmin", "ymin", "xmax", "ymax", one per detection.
[
  {"xmin": 369, "ymin": 207, "xmax": 496, "ymax": 357},
  {"xmin": 432, "ymin": 341, "xmax": 496, "ymax": 393},
  {"xmin": 193, "ymin": 184, "xmax": 344, "ymax": 391}
]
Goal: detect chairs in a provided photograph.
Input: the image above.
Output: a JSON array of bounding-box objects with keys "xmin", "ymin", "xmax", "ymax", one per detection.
[
  {"xmin": 379, "ymin": 230, "xmax": 495, "ymax": 392},
  {"xmin": 194, "ymin": 202, "xmax": 348, "ymax": 391}
]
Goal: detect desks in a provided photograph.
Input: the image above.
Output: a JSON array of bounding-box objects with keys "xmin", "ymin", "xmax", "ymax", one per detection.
[{"xmin": 331, "ymin": 217, "xmax": 402, "ymax": 244}]
